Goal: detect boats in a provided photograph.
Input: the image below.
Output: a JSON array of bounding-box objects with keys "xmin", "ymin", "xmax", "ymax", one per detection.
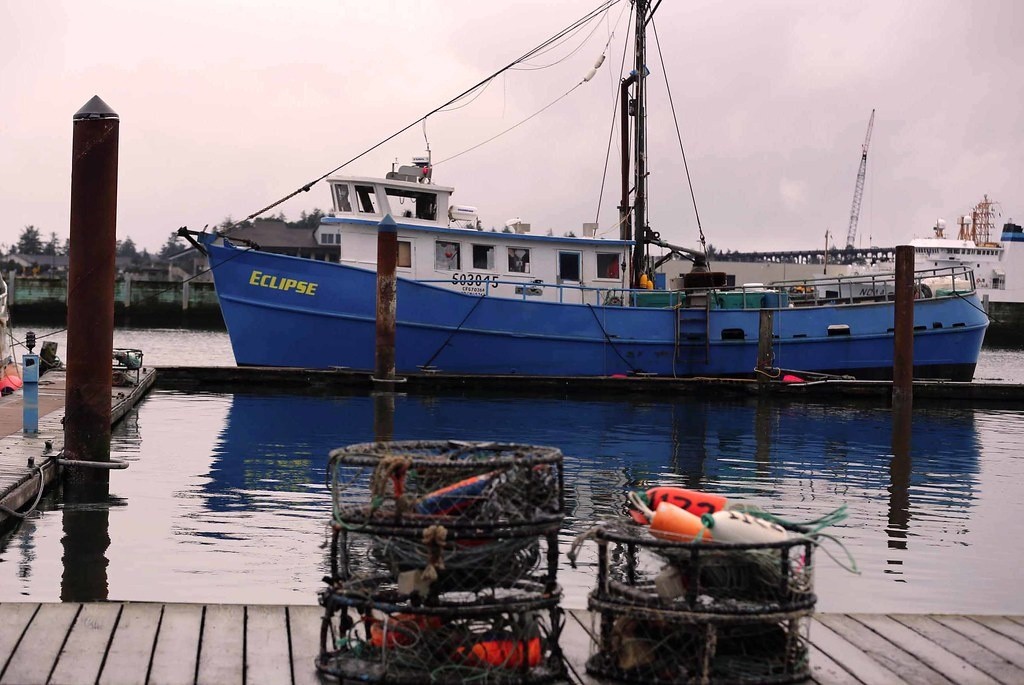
[{"xmin": 180, "ymin": 1, "xmax": 998, "ymax": 380}]
